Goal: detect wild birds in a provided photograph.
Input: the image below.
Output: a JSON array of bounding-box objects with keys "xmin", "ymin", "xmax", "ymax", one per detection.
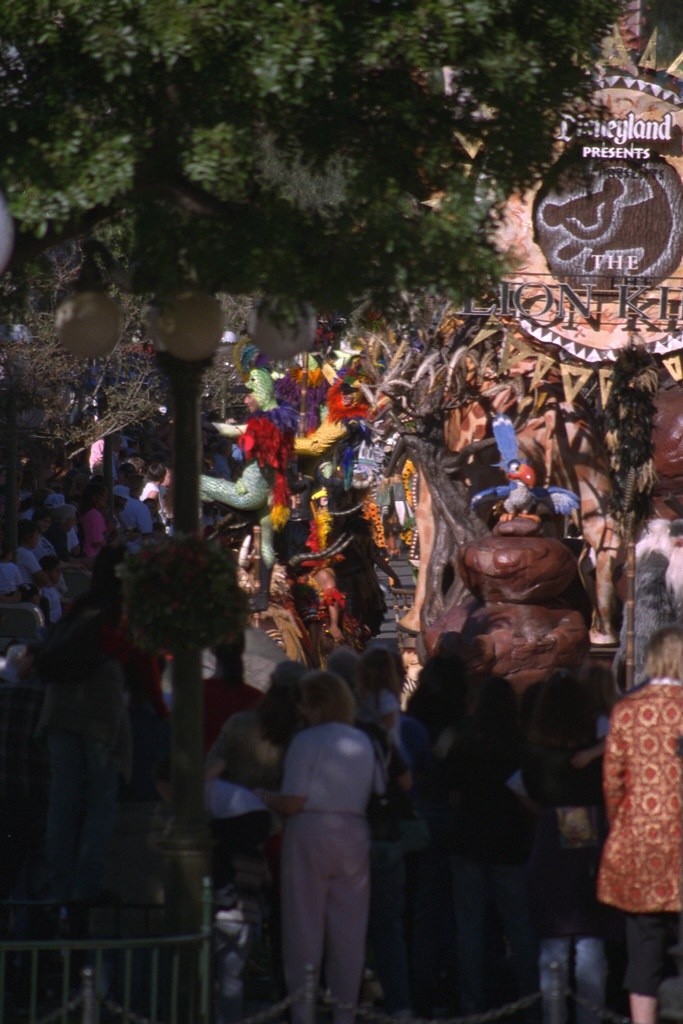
[{"xmin": 470, "ymin": 413, "xmax": 582, "ymax": 524}]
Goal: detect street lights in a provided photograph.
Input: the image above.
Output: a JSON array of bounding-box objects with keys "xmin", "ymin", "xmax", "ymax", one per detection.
[{"xmin": 53, "ymin": 278, "xmax": 320, "ymax": 1023}]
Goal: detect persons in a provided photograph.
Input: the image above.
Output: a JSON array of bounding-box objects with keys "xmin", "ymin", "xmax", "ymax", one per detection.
[
  {"xmin": 251, "ymin": 671, "xmax": 386, "ymax": 1024},
  {"xmin": 0, "ymin": 315, "xmax": 683, "ymax": 1024},
  {"xmin": 597, "ymin": 628, "xmax": 683, "ymax": 1024}
]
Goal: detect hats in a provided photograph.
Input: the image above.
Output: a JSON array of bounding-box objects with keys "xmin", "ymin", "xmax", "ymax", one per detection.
[
  {"xmin": 113, "ymin": 486, "xmax": 133, "ymax": 500},
  {"xmin": 44, "ymin": 494, "xmax": 65, "ymax": 509},
  {"xmin": 272, "ymin": 661, "xmax": 307, "ymax": 687}
]
